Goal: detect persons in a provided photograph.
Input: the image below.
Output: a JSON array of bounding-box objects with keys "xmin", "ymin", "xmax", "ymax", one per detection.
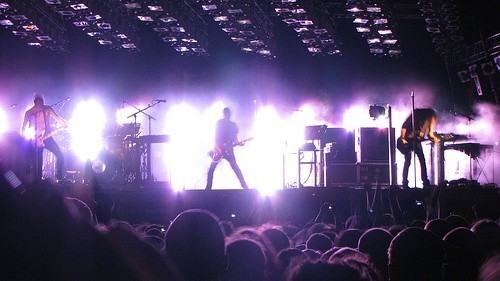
[
  {"xmin": 0, "ymin": 160, "xmax": 500, "ymax": 281},
  {"xmin": 400, "ymin": 107, "xmax": 440, "ymax": 189},
  {"xmin": 205, "ymin": 107, "xmax": 249, "ymax": 191},
  {"xmin": 21, "ymin": 93, "xmax": 68, "ymax": 183}
]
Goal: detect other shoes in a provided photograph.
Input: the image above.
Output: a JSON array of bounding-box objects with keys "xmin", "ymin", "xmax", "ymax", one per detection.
[
  {"xmin": 241, "ymin": 183, "xmax": 248, "ymax": 189},
  {"xmin": 206, "ymin": 183, "xmax": 212, "ymax": 190}
]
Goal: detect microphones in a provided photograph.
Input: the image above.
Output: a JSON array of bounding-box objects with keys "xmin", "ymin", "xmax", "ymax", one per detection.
[
  {"xmin": 63, "ymin": 98, "xmax": 70, "ymax": 102},
  {"xmin": 156, "ymin": 99, "xmax": 166, "ymax": 102},
  {"xmin": 122, "ymin": 98, "xmax": 124, "ymax": 108}
]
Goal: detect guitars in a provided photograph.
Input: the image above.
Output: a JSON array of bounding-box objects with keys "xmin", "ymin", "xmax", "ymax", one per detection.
[
  {"xmin": 31, "ymin": 125, "xmax": 67, "ymax": 149},
  {"xmin": 207, "ymin": 136, "xmax": 253, "ymax": 162},
  {"xmin": 396, "ymin": 133, "xmax": 453, "ymax": 155}
]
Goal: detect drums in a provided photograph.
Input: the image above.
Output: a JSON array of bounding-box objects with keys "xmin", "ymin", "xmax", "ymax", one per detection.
[{"xmin": 85, "ymin": 148, "xmax": 119, "ymax": 184}]
